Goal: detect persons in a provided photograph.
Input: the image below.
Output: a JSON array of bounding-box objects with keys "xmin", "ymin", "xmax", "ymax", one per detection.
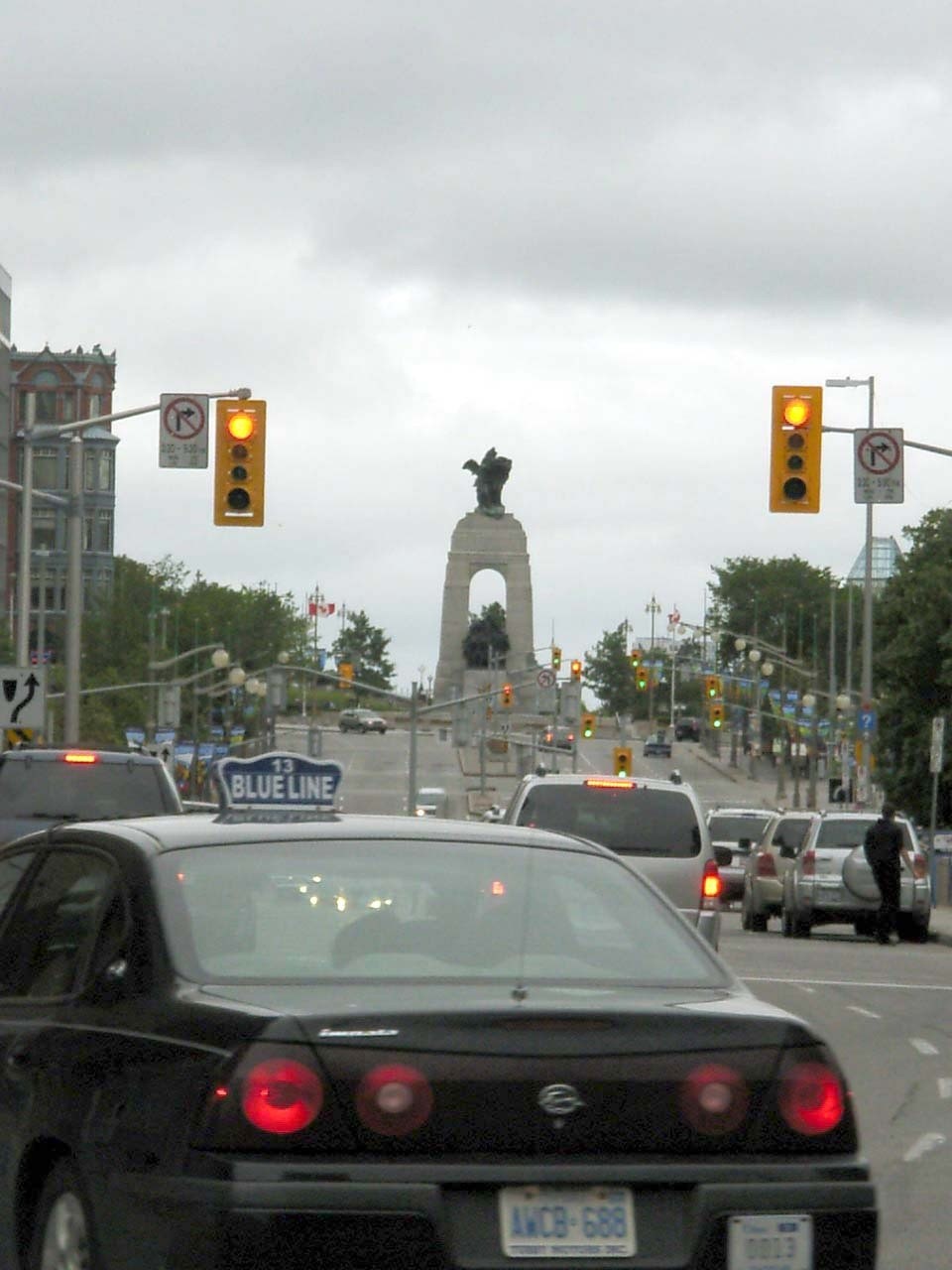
[{"xmin": 864, "ymin": 802, "xmax": 920, "ymax": 945}]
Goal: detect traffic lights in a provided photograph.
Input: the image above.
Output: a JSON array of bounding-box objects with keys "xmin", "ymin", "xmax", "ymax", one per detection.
[
  {"xmin": 503, "ymin": 687, "xmax": 510, "ymax": 704},
  {"xmin": 572, "ymin": 663, "xmax": 580, "ymax": 680},
  {"xmin": 214, "ymin": 399, "xmax": 265, "ymax": 527},
  {"xmin": 583, "ymin": 718, "xmax": 594, "ymax": 737},
  {"xmin": 769, "ymin": 386, "xmax": 823, "ymax": 513},
  {"xmin": 554, "ymin": 651, "xmax": 560, "ymax": 668}
]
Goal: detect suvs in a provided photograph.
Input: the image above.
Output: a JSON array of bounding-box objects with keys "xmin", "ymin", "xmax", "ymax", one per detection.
[
  {"xmin": 675, "ymin": 716, "xmax": 710, "ymax": 743},
  {"xmin": 337, "ymin": 707, "xmax": 387, "ymax": 734},
  {"xmin": 2, "ymin": 738, "xmax": 184, "ymax": 839},
  {"xmin": 706, "ymin": 802, "xmax": 932, "ymax": 943},
  {"xmin": 499, "ymin": 762, "xmax": 721, "ymax": 952}
]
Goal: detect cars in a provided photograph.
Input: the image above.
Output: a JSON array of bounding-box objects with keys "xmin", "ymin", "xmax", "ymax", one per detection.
[
  {"xmin": 413, "ymin": 787, "xmax": 451, "ymax": 817},
  {"xmin": 0, "ymin": 816, "xmax": 883, "ymax": 1270},
  {"xmin": 643, "ymin": 730, "xmax": 673, "ymax": 757}
]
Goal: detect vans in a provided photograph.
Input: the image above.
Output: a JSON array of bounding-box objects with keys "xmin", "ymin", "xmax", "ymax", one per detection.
[{"xmin": 538, "ymin": 724, "xmax": 576, "ymax": 751}]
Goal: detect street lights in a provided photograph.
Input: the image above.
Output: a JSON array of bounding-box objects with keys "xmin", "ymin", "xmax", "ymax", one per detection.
[
  {"xmin": 145, "ymin": 640, "xmax": 300, "ymax": 766},
  {"xmin": 825, "ymin": 376, "xmax": 877, "ymax": 811},
  {"xmin": 614, "ymin": 587, "xmax": 889, "ymax": 809}
]
